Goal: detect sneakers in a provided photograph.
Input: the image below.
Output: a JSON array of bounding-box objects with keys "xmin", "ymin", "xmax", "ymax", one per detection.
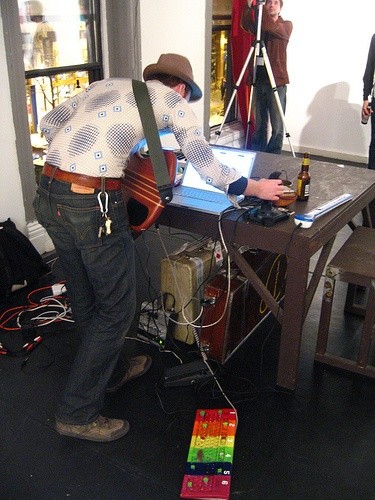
[
  {"xmin": 57, "ymin": 413, "xmax": 129, "ymax": 444},
  {"xmin": 104, "ymin": 352, "xmax": 152, "ymax": 393}
]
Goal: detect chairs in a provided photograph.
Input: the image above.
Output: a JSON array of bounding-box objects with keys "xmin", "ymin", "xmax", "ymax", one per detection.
[{"xmin": 314, "ymin": 225, "xmax": 375, "ymax": 379}]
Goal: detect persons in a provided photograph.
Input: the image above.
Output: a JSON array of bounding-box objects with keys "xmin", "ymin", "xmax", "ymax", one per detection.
[
  {"xmin": 361, "ymin": 34, "xmax": 375, "ymax": 172},
  {"xmin": 32, "ymin": 53, "xmax": 285, "ymax": 441},
  {"xmin": 241, "ymin": 0, "xmax": 294, "ymax": 153}
]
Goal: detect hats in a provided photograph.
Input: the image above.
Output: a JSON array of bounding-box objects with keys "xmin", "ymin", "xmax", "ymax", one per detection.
[{"xmin": 142, "ymin": 53, "xmax": 202, "ymax": 102}]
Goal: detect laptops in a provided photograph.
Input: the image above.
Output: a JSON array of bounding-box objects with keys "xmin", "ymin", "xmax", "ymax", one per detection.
[
  {"xmin": 132, "ymin": 128, "xmax": 180, "ymax": 155},
  {"xmin": 166, "ymin": 144, "xmax": 258, "ymax": 215}
]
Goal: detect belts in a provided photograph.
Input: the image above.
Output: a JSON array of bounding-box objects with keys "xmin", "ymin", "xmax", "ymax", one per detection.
[{"xmin": 41, "ymin": 162, "xmax": 124, "ymax": 191}]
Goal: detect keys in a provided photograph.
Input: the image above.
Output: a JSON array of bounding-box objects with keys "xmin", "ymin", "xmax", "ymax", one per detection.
[{"xmin": 97, "ymin": 224, "xmax": 105, "ymax": 240}]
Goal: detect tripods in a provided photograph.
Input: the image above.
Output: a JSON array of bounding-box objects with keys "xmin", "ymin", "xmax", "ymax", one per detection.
[{"xmin": 215, "ymin": 0, "xmax": 296, "ymax": 158}]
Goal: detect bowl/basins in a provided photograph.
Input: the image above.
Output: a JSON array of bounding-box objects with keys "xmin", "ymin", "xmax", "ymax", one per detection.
[{"xmin": 274, "ymin": 178, "xmax": 292, "ymax": 190}]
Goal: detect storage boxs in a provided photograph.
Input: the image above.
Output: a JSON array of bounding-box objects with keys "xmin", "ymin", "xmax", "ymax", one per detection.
[{"xmin": 160, "ymin": 235, "xmax": 288, "ymax": 367}]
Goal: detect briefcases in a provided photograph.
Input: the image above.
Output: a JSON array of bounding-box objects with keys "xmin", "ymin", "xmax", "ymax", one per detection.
[
  {"xmin": 198, "ymin": 248, "xmax": 288, "ymax": 365},
  {"xmin": 161, "ymin": 235, "xmax": 241, "ymax": 345}
]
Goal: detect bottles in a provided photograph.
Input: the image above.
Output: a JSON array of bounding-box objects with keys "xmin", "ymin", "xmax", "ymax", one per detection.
[{"xmin": 296, "ymin": 152, "xmax": 311, "ymax": 201}]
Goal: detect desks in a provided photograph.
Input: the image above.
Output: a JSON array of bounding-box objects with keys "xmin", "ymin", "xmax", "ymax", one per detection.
[{"xmin": 152, "ymin": 129, "xmax": 375, "ymax": 390}]
